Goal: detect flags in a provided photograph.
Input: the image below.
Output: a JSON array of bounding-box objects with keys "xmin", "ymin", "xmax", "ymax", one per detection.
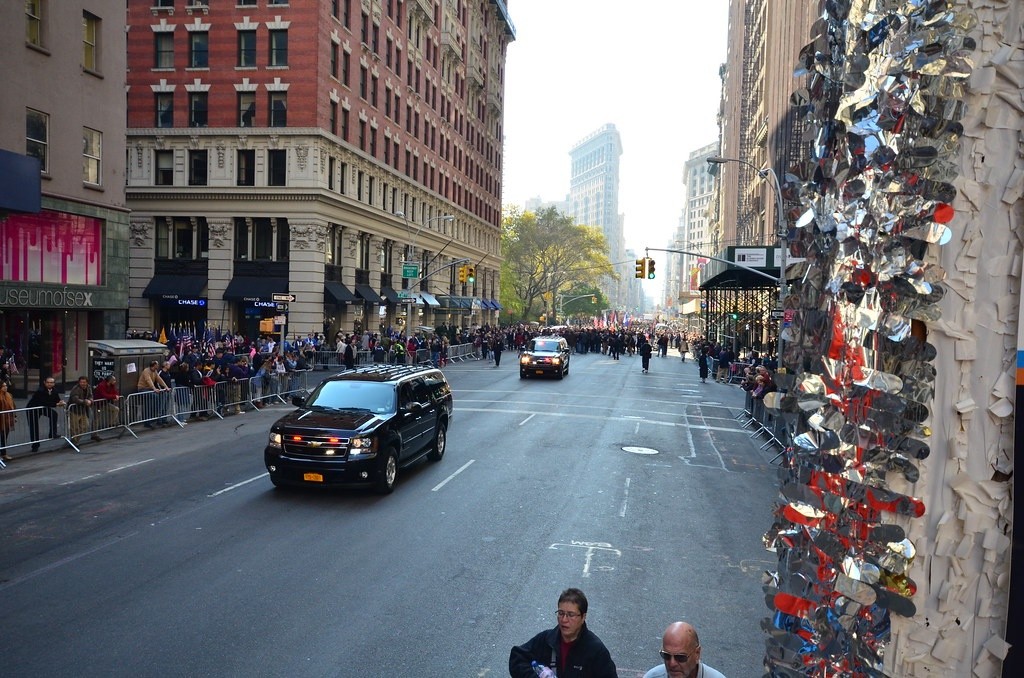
[
  {"xmin": 611, "ymin": 311, "xmax": 628, "ymax": 327},
  {"xmin": 169, "ymin": 326, "xmax": 237, "ymax": 360},
  {"xmin": 594, "ymin": 316, "xmax": 603, "ymax": 327},
  {"xmin": 9, "ymin": 354, "xmax": 19, "ymax": 374}
]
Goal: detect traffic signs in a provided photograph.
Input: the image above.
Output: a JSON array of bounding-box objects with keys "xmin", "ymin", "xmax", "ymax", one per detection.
[
  {"xmin": 400, "ymin": 298, "xmax": 417, "ymax": 304},
  {"xmin": 272, "ymin": 293, "xmax": 297, "ymax": 312},
  {"xmin": 770, "ymin": 309, "xmax": 784, "ymax": 319}
]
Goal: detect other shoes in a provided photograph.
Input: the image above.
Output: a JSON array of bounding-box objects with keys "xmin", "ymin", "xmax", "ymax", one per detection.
[
  {"xmin": 236, "ymin": 411, "xmax": 246, "ymax": 414},
  {"xmin": 48, "ymin": 432, "xmax": 61, "ymax": 438},
  {"xmin": 254, "ymin": 396, "xmax": 293, "ymax": 410},
  {"xmin": 145, "ymin": 423, "xmax": 153, "ymax": 429},
  {"xmin": 3, "ymin": 455, "xmax": 13, "ymax": 461},
  {"xmin": 32, "ymin": 447, "xmax": 38, "ymax": 452},
  {"xmin": 190, "ymin": 415, "xmax": 199, "ymax": 419},
  {"xmin": 90, "ymin": 435, "xmax": 102, "ymax": 442},
  {"xmin": 200, "ymin": 413, "xmax": 210, "ymax": 417},
  {"xmin": 642, "ymin": 369, "xmax": 648, "ymax": 374}
]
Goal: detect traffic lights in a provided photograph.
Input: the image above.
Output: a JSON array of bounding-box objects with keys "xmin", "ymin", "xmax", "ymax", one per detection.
[
  {"xmin": 458, "ymin": 266, "xmax": 468, "ymax": 283},
  {"xmin": 468, "ymin": 268, "xmax": 475, "ymax": 283},
  {"xmin": 592, "ymin": 297, "xmax": 598, "ymax": 304},
  {"xmin": 648, "ymin": 258, "xmax": 655, "ymax": 279},
  {"xmin": 732, "ymin": 313, "xmax": 738, "ymax": 319},
  {"xmin": 636, "ymin": 258, "xmax": 646, "ymax": 278},
  {"xmin": 540, "ymin": 317, "xmax": 546, "ymax": 321}
]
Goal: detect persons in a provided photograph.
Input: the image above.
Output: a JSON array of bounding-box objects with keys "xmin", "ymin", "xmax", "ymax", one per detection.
[
  {"xmin": 26, "ymin": 376, "xmax": 67, "ymax": 452},
  {"xmin": 0, "ymin": 380, "xmax": 17, "ymax": 459},
  {"xmin": 643, "ymin": 622, "xmax": 725, "ymax": 678},
  {"xmin": 91, "ymin": 374, "xmax": 119, "ymax": 441},
  {"xmin": 509, "ymin": 587, "xmax": 618, "ymax": 678},
  {"xmin": 137, "ymin": 335, "xmax": 314, "ymax": 426},
  {"xmin": 66, "ymin": 376, "xmax": 94, "ymax": 449},
  {"xmin": 414, "ymin": 322, "xmax": 503, "ymax": 367},
  {"xmin": 669, "ymin": 330, "xmax": 735, "ymax": 384},
  {"xmin": 503, "ymin": 323, "xmax": 589, "ymax": 355},
  {"xmin": 589, "ymin": 326, "xmax": 669, "ymax": 373},
  {"xmin": 292, "ymin": 328, "xmax": 418, "ymax": 369},
  {"xmin": 0, "ymin": 343, "xmax": 7, "ymax": 383},
  {"xmin": 735, "ymin": 336, "xmax": 776, "ymax": 428}
]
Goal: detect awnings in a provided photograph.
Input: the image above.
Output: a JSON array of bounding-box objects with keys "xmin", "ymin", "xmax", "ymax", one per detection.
[
  {"xmin": 380, "ymin": 285, "xmax": 403, "ymax": 305},
  {"xmin": 412, "ymin": 290, "xmax": 441, "ymax": 307},
  {"xmin": 223, "ymin": 276, "xmax": 288, "ymax": 302},
  {"xmin": 481, "ymin": 298, "xmax": 503, "ymax": 310},
  {"xmin": 142, "ymin": 274, "xmax": 207, "ymax": 300},
  {"xmin": 325, "ymin": 281, "xmax": 359, "ymax": 304},
  {"xmin": 355, "ymin": 283, "xmax": 386, "ymax": 305}
]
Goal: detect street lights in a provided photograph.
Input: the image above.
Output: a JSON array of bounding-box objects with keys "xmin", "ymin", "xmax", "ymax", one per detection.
[
  {"xmin": 546, "ymin": 271, "xmax": 566, "ymax": 325},
  {"xmin": 706, "ymin": 154, "xmax": 788, "ymax": 369},
  {"xmin": 675, "ymin": 240, "xmax": 703, "ymax": 336},
  {"xmin": 394, "ymin": 210, "xmax": 455, "ymax": 364}
]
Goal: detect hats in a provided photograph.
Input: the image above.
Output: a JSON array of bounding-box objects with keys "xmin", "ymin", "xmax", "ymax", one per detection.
[{"xmin": 192, "ymin": 344, "xmax": 199, "ymax": 349}]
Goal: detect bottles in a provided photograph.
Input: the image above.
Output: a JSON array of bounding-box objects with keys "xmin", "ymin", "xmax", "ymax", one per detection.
[{"xmin": 531, "ymin": 661, "xmax": 557, "ymax": 678}]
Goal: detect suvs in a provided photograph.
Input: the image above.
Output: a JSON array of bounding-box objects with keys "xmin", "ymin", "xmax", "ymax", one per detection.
[
  {"xmin": 264, "ymin": 363, "xmax": 453, "ymax": 495},
  {"xmin": 519, "ymin": 335, "xmax": 571, "ymax": 379}
]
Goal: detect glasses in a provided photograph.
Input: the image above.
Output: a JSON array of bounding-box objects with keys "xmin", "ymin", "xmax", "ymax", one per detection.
[
  {"xmin": 659, "ymin": 647, "xmax": 697, "ymax": 662},
  {"xmin": 554, "ymin": 610, "xmax": 583, "ymax": 620}
]
